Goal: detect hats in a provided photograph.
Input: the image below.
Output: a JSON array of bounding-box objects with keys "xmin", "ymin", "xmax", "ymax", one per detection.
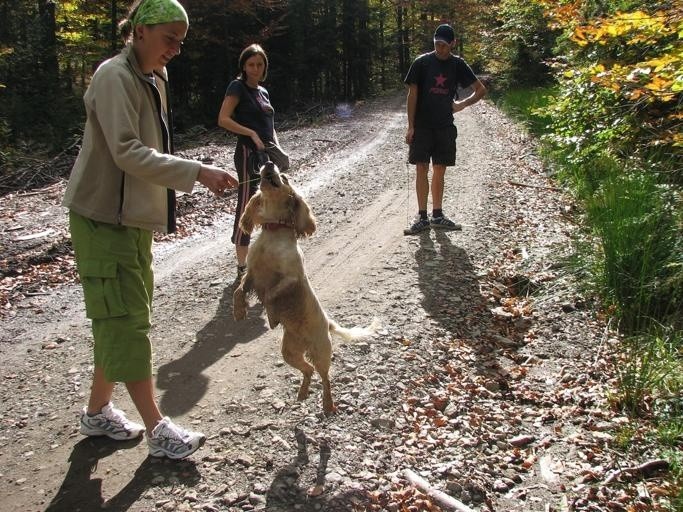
[{"xmin": 434, "ymin": 25, "xmax": 455, "ymax": 46}]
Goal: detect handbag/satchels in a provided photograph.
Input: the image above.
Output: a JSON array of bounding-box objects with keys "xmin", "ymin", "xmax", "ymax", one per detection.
[{"xmin": 265, "ymin": 142, "xmax": 289, "ymax": 173}]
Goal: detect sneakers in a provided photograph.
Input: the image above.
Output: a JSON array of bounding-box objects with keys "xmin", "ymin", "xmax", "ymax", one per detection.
[
  {"xmin": 80, "ymin": 401, "xmax": 146, "ymax": 440},
  {"xmin": 238, "ymin": 266, "xmax": 247, "ymax": 282},
  {"xmin": 431, "ymin": 216, "xmax": 462, "ymax": 231},
  {"xmin": 404, "ymin": 218, "xmax": 430, "ymax": 234},
  {"xmin": 146, "ymin": 417, "xmax": 206, "ymax": 458}
]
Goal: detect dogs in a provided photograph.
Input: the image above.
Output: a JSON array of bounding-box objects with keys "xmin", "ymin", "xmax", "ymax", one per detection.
[{"xmin": 232, "ymin": 160, "xmax": 381, "ymax": 418}]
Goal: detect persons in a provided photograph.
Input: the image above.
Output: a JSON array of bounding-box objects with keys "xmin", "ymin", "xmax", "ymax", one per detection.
[
  {"xmin": 218, "ymin": 44, "xmax": 284, "ymax": 284},
  {"xmin": 403, "ymin": 23, "xmax": 487, "ymax": 235},
  {"xmin": 61, "ymin": 0, "xmax": 240, "ymax": 460}
]
